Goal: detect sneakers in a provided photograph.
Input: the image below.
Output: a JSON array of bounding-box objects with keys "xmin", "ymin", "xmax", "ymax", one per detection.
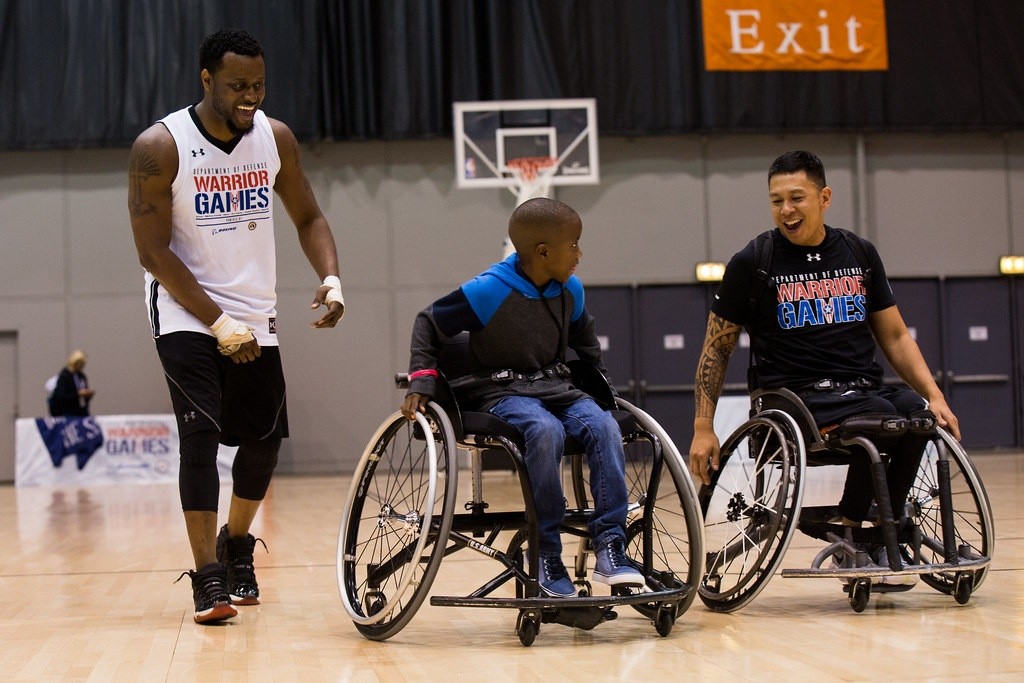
[
  {"xmin": 869, "ymin": 542, "xmax": 920, "ymax": 586},
  {"xmin": 525, "ymin": 547, "xmax": 576, "ymax": 598},
  {"xmin": 215, "ymin": 523, "xmax": 268, "ymax": 604},
  {"xmin": 826, "ymin": 517, "xmax": 883, "ymax": 586},
  {"xmin": 173, "ymin": 563, "xmax": 238, "ymax": 623},
  {"xmin": 592, "ymin": 537, "xmax": 645, "ymax": 586}
]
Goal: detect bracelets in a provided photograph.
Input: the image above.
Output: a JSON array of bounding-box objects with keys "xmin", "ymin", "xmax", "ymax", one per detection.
[{"xmin": 407, "ymin": 369, "xmax": 438, "ymax": 382}]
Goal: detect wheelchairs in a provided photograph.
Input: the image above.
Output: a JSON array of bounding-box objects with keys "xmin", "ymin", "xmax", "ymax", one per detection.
[
  {"xmin": 688, "ymin": 340, "xmax": 997, "ymax": 614},
  {"xmin": 336, "ymin": 330, "xmax": 707, "ymax": 648}
]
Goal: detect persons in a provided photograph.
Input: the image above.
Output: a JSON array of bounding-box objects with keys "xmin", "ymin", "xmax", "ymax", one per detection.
[
  {"xmin": 44, "ymin": 350, "xmax": 95, "ymax": 416},
  {"xmin": 400, "ymin": 198, "xmax": 647, "ymax": 598},
  {"xmin": 688, "ymin": 151, "xmax": 960, "ymax": 558},
  {"xmin": 127, "ymin": 26, "xmax": 344, "ymax": 625}
]
